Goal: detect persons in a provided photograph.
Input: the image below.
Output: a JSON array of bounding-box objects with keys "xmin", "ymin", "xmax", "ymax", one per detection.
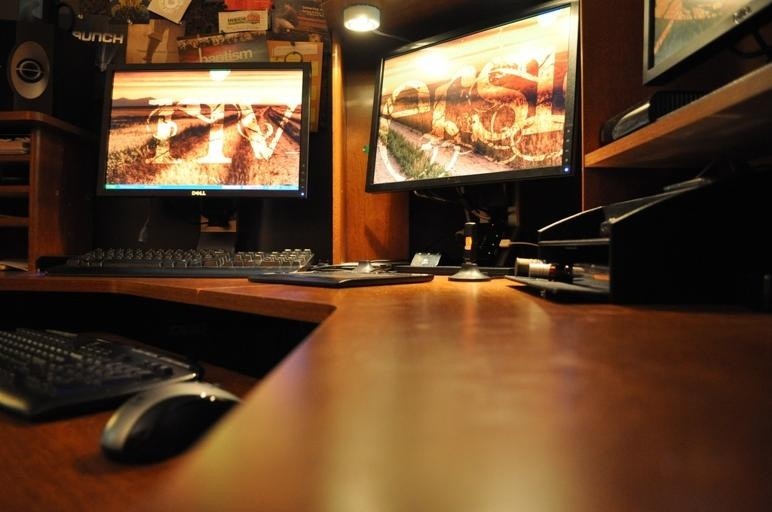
[{"xmin": 273, "ymin": 4, "xmax": 300, "ymax": 34}]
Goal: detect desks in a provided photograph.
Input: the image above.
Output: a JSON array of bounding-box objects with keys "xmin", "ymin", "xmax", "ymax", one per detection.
[{"xmin": 0, "ymin": 262, "xmax": 771, "ymax": 510}]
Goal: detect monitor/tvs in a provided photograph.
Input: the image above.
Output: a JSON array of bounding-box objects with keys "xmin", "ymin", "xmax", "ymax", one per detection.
[
  {"xmin": 365, "ymin": 0, "xmax": 580, "ymax": 276},
  {"xmin": 96, "ymin": 62, "xmax": 312, "ymax": 252},
  {"xmin": 643, "ymin": 0, "xmax": 772, "ymax": 86}
]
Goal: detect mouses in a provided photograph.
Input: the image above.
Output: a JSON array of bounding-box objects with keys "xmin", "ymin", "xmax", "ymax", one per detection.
[{"xmin": 100, "ymin": 381, "xmax": 241, "ymax": 464}]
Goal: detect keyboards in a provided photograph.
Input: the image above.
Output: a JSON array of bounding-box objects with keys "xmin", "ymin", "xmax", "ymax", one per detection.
[
  {"xmin": 0, "ymin": 328, "xmax": 206, "ymax": 422},
  {"xmin": 47, "ymin": 247, "xmax": 316, "ymax": 278}
]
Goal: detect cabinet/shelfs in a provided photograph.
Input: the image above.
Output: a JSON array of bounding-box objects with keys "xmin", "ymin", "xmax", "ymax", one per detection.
[{"xmin": 0, "ymin": 112, "xmax": 101, "ymax": 273}]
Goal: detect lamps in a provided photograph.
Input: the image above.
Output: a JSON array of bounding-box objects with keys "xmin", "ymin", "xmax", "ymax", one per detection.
[{"xmin": 342, "ymin": 4, "xmax": 413, "ymax": 44}]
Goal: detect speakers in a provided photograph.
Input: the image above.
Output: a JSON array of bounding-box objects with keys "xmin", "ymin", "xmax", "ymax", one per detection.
[{"xmin": 0, "ymin": 18, "xmax": 56, "ymax": 115}]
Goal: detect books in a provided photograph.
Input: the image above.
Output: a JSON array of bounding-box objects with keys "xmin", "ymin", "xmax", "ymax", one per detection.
[
  {"xmin": 0, "ymin": 136, "xmax": 31, "ymax": 155},
  {"xmin": 0, "ymin": 259, "xmax": 27, "ymax": 273}
]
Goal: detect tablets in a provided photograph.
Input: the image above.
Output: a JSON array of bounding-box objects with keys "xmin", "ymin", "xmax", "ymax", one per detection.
[{"xmin": 248, "ymin": 270, "xmax": 433, "ymax": 288}]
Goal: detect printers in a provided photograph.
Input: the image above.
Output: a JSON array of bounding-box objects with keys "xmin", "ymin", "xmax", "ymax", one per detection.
[{"xmin": 504, "ymin": 167, "xmax": 772, "ymax": 306}]
